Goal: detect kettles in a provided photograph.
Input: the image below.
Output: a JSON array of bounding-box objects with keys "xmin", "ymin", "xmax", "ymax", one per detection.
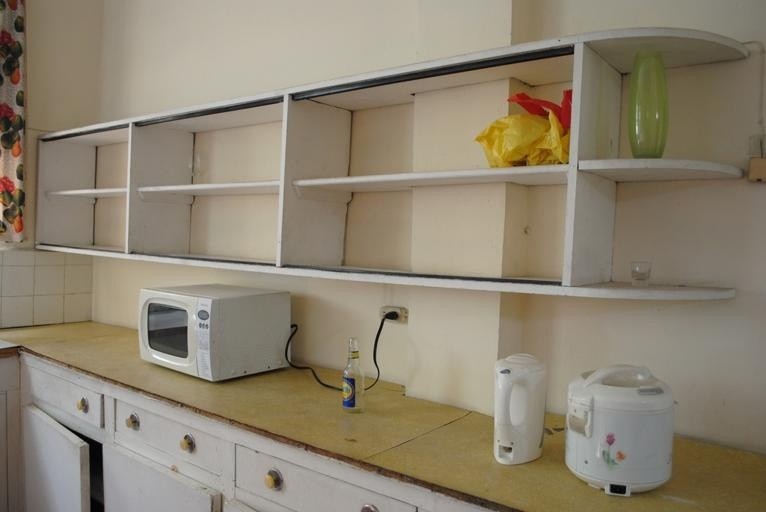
[{"xmin": 492, "ymin": 352, "xmax": 548, "ymax": 467}]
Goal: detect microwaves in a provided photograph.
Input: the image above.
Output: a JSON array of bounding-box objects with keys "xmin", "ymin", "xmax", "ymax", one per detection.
[{"xmin": 139, "ymin": 284, "xmax": 291, "ymax": 383}]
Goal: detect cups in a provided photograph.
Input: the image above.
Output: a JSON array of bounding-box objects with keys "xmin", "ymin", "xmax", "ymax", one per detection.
[{"xmin": 629, "ymin": 258, "xmax": 651, "ymax": 286}]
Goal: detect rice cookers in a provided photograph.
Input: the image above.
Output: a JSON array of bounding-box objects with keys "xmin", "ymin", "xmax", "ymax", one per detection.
[{"xmin": 564, "ymin": 365, "xmax": 676, "ymax": 497}]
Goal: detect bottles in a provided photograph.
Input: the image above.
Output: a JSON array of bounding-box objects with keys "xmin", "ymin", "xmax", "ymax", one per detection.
[{"xmin": 342, "ymin": 337, "xmax": 363, "ymax": 413}]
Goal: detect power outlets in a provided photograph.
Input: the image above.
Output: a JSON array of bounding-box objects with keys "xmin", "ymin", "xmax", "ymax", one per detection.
[{"xmin": 379, "ymin": 306, "xmax": 407, "ymax": 322}]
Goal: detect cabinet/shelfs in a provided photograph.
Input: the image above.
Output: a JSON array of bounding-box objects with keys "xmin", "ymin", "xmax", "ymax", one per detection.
[
  {"xmin": 18, "ymin": 350, "xmax": 224, "ymax": 511},
  {"xmin": 224, "ymin": 421, "xmax": 482, "ymax": 512},
  {"xmin": 36, "ymin": 29, "xmax": 749, "ymax": 301}
]
